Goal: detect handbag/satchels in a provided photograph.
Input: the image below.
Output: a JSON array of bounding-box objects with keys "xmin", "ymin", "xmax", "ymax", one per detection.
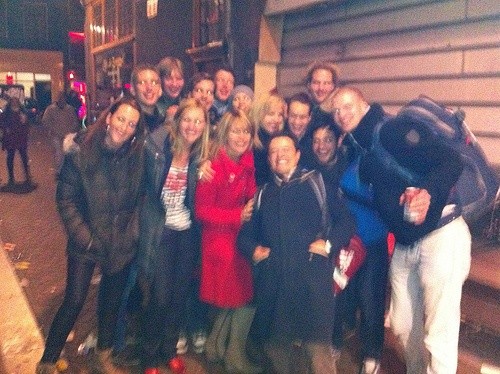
[{"xmin": 333, "ymin": 234, "xmax": 364, "ymax": 296}]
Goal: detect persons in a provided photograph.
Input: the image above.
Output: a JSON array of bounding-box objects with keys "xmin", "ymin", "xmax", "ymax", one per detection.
[
  {"xmin": 0, "ymin": 55, "xmax": 390, "ymax": 374},
  {"xmin": 332, "ymin": 85, "xmax": 471, "ymax": 374}
]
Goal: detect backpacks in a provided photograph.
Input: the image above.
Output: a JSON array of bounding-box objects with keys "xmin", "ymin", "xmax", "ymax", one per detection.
[{"xmin": 371, "ymin": 94, "xmax": 500, "ymax": 224}]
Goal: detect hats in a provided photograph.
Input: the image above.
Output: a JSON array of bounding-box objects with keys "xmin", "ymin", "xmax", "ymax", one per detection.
[{"xmin": 230, "ymin": 84, "xmax": 254, "ymax": 102}]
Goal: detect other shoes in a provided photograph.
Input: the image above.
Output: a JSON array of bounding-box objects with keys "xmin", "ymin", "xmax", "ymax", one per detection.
[
  {"xmin": 166, "ymin": 356, "xmax": 188, "ymax": 374},
  {"xmin": 36, "ymin": 360, "xmax": 57, "ymax": 374},
  {"xmin": 361, "ymin": 357, "xmax": 381, "ymax": 374},
  {"xmin": 190, "ymin": 331, "xmax": 207, "ymax": 354},
  {"xmin": 176, "ymin": 332, "xmax": 189, "ymax": 354},
  {"xmin": 95, "ymin": 348, "xmax": 123, "ymax": 374},
  {"xmin": 144, "ymin": 367, "xmax": 158, "ymax": 374}
]
